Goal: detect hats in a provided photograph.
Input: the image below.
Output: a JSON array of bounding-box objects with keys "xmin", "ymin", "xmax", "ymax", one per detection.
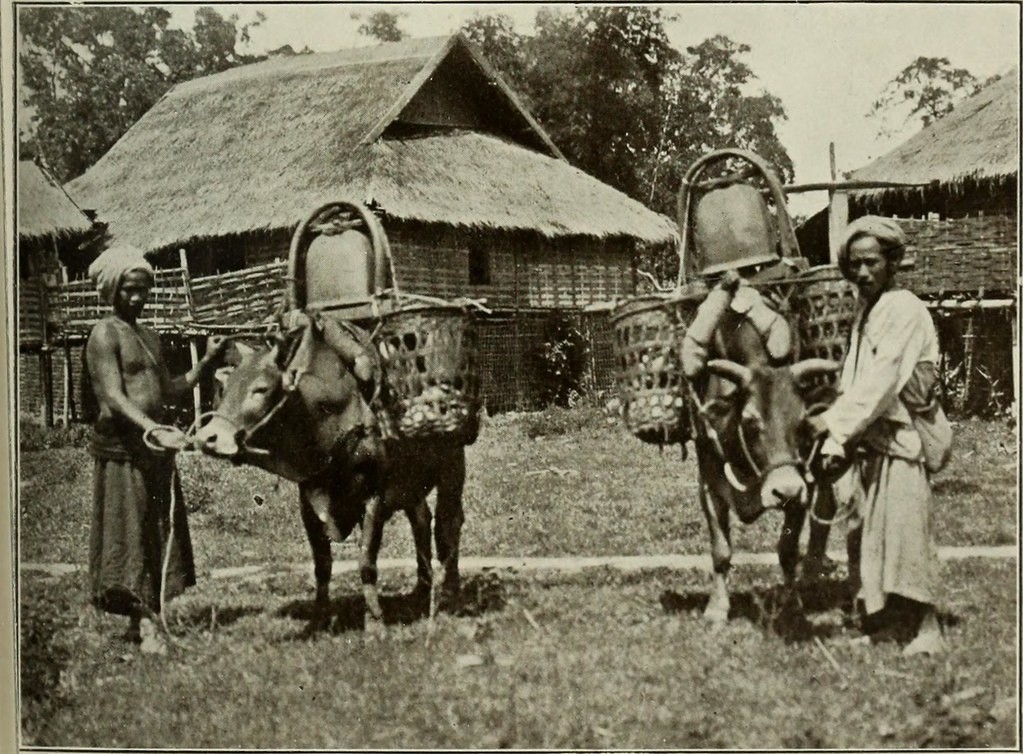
[
  {"xmin": 838, "ymin": 217, "xmax": 907, "ymax": 277},
  {"xmin": 88, "ymin": 245, "xmax": 153, "ymax": 307}
]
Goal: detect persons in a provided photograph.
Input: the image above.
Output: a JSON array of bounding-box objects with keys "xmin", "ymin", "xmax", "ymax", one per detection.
[
  {"xmin": 85, "ymin": 243, "xmax": 238, "ymax": 651},
  {"xmin": 805, "ymin": 216, "xmax": 956, "ymax": 654}
]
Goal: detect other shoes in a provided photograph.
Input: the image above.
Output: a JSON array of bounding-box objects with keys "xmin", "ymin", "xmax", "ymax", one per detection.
[
  {"xmin": 136, "ymin": 631, "xmax": 169, "ymax": 658},
  {"xmin": 903, "ymin": 627, "xmax": 946, "ymax": 658}
]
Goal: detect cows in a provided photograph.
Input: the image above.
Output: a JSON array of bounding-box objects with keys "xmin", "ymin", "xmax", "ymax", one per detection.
[
  {"xmin": 691, "ymin": 270, "xmax": 838, "ymax": 628},
  {"xmin": 195, "ymin": 331, "xmax": 466, "ymax": 632}
]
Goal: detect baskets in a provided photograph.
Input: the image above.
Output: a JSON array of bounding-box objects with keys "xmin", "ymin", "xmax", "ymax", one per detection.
[
  {"xmin": 367, "ymin": 306, "xmax": 481, "ymax": 443},
  {"xmin": 611, "ymin": 300, "xmax": 691, "ymax": 447},
  {"xmin": 791, "ymin": 268, "xmax": 861, "ymax": 402}
]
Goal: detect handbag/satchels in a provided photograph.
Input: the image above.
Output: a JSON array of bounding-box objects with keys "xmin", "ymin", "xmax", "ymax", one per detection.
[{"xmin": 907, "ymin": 389, "xmax": 953, "ymax": 472}]
